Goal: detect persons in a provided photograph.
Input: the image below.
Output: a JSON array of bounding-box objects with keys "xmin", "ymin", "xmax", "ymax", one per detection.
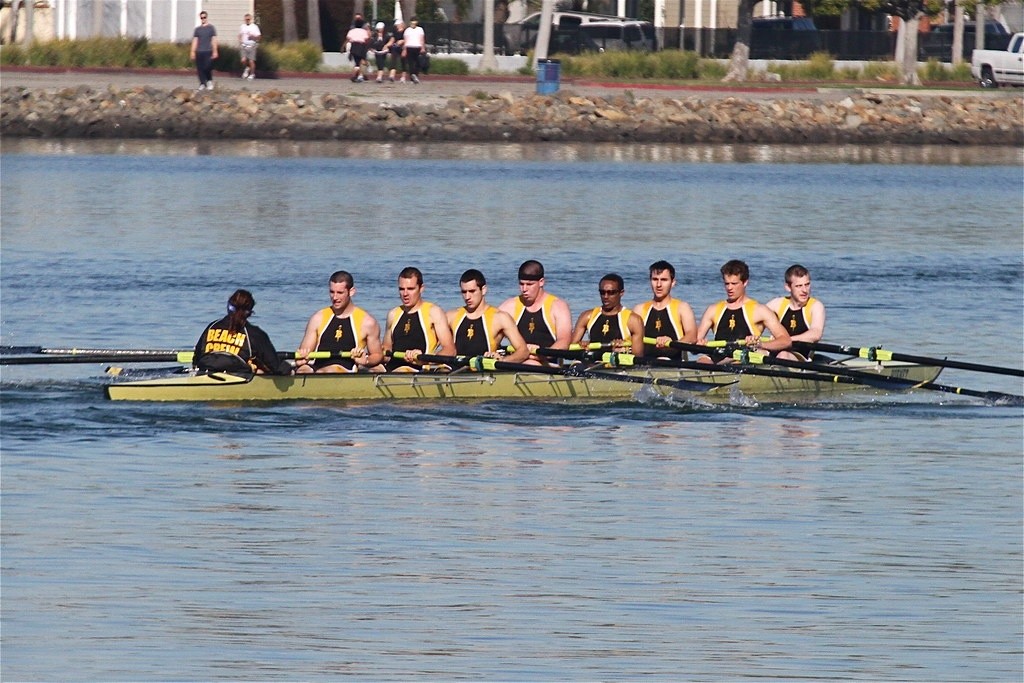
[
  {"xmin": 697, "ymin": 259, "xmax": 792, "ymax": 364},
  {"xmin": 435, "ymin": 269, "xmax": 530, "ymax": 372},
  {"xmin": 493, "ymin": 260, "xmax": 571, "ymax": 367},
  {"xmin": 757, "ymin": 264, "xmax": 825, "ymax": 362},
  {"xmin": 632, "ymin": 260, "xmax": 698, "ymax": 361},
  {"xmin": 571, "ymin": 274, "xmax": 645, "ymax": 364},
  {"xmin": 341, "ymin": 13, "xmax": 426, "ymax": 83},
  {"xmin": 192, "ymin": 289, "xmax": 293, "ymax": 375},
  {"xmin": 365, "ymin": 266, "xmax": 456, "ymax": 372},
  {"xmin": 239, "ymin": 14, "xmax": 261, "ymax": 80},
  {"xmin": 296, "ymin": 271, "xmax": 382, "ymax": 372},
  {"xmin": 191, "ymin": 11, "xmax": 217, "ymax": 91}
]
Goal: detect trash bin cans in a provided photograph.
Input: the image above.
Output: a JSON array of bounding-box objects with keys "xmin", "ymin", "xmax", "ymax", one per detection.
[{"xmin": 535, "ymin": 58, "xmax": 560, "ymax": 95}]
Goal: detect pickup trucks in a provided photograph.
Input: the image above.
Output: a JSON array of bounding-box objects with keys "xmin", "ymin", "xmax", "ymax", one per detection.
[{"xmin": 970, "ymin": 31, "xmax": 1024, "ymax": 88}]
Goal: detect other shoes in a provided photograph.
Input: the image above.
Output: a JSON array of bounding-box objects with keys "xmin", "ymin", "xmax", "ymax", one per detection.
[
  {"xmin": 389, "ymin": 76, "xmax": 394, "ymax": 81},
  {"xmin": 243, "ymin": 69, "xmax": 249, "ymax": 77},
  {"xmin": 375, "ymin": 77, "xmax": 382, "ymax": 83},
  {"xmin": 199, "ymin": 84, "xmax": 206, "ymax": 90},
  {"xmin": 207, "ymin": 82, "xmax": 213, "ymax": 91},
  {"xmin": 412, "ymin": 75, "xmax": 420, "ymax": 83},
  {"xmin": 400, "ymin": 78, "xmax": 407, "ymax": 83},
  {"xmin": 351, "ymin": 76, "xmax": 361, "ymax": 82},
  {"xmin": 248, "ymin": 74, "xmax": 255, "ymax": 80}
]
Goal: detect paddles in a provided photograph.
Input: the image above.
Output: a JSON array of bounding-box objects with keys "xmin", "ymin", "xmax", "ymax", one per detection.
[
  {"xmin": 499, "ymin": 346, "xmax": 930, "ymax": 392},
  {"xmin": 568, "ymin": 340, "xmax": 632, "ymax": 351},
  {"xmin": 642, "ymin": 336, "xmax": 1024, "ymax": 406},
  {"xmin": 759, "ymin": 336, "xmax": 1024, "ymax": 378},
  {"xmin": 706, "ymin": 339, "xmax": 748, "ymax": 347},
  {"xmin": 105, "ymin": 362, "xmax": 298, "ymax": 377},
  {"xmin": 382, "ymin": 349, "xmax": 740, "ymax": 392},
  {"xmin": 0, "ymin": 345, "xmax": 351, "ymax": 358},
  {"xmin": 0, "ymin": 357, "xmax": 193, "ymax": 365}
]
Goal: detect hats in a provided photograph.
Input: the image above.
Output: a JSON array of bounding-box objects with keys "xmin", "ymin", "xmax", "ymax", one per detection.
[
  {"xmin": 375, "ymin": 22, "xmax": 385, "ymax": 29},
  {"xmin": 393, "ymin": 20, "xmax": 404, "ymax": 26}
]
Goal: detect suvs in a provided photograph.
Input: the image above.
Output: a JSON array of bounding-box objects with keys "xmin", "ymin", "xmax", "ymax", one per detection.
[
  {"xmin": 727, "ymin": 14, "xmax": 819, "ymax": 59},
  {"xmin": 918, "ymin": 17, "xmax": 1012, "ymax": 64},
  {"xmin": 496, "ymin": 9, "xmax": 656, "ymax": 57}
]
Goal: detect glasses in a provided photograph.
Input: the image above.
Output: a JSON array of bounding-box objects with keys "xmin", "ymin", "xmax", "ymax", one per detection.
[{"xmin": 201, "ymin": 17, "xmax": 207, "ymax": 19}]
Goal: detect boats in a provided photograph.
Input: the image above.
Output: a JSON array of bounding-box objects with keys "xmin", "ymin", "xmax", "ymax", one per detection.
[{"xmin": 101, "ymin": 351, "xmax": 950, "ymax": 401}]
